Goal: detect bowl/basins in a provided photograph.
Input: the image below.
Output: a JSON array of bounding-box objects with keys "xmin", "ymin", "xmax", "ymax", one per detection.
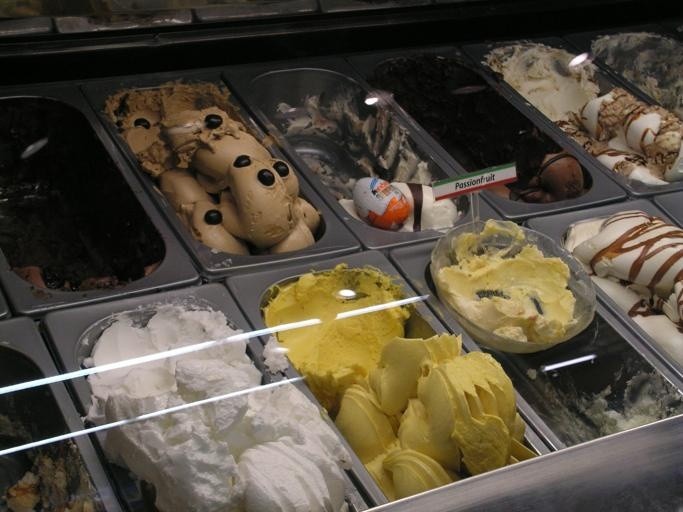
[{"xmin": 430, "ymin": 218, "xmax": 596, "ymax": 355}]
